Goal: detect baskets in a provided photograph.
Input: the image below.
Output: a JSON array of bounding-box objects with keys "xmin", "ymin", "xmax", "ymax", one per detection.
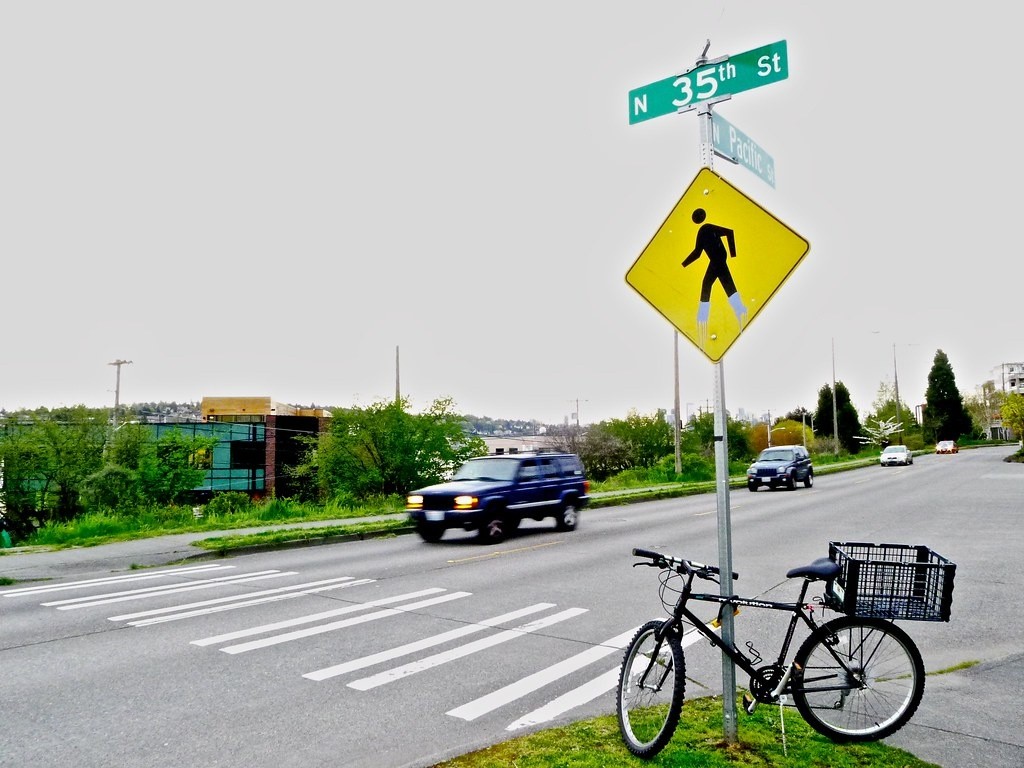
[{"xmin": 829, "ymin": 541, "xmax": 957, "ymax": 622}]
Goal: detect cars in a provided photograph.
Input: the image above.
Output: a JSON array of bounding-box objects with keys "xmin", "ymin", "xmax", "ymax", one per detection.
[
  {"xmin": 880, "ymin": 445, "xmax": 914, "ymax": 466},
  {"xmin": 935, "ymin": 441, "xmax": 959, "ymax": 454}
]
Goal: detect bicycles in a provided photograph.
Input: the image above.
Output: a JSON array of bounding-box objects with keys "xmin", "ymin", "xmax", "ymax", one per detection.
[{"xmin": 615, "ymin": 547, "xmax": 926, "ymax": 758}]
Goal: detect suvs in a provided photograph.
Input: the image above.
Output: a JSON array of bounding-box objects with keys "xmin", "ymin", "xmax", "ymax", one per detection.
[
  {"xmin": 401, "ymin": 447, "xmax": 591, "ymax": 545},
  {"xmin": 746, "ymin": 445, "xmax": 813, "ymax": 492}
]
